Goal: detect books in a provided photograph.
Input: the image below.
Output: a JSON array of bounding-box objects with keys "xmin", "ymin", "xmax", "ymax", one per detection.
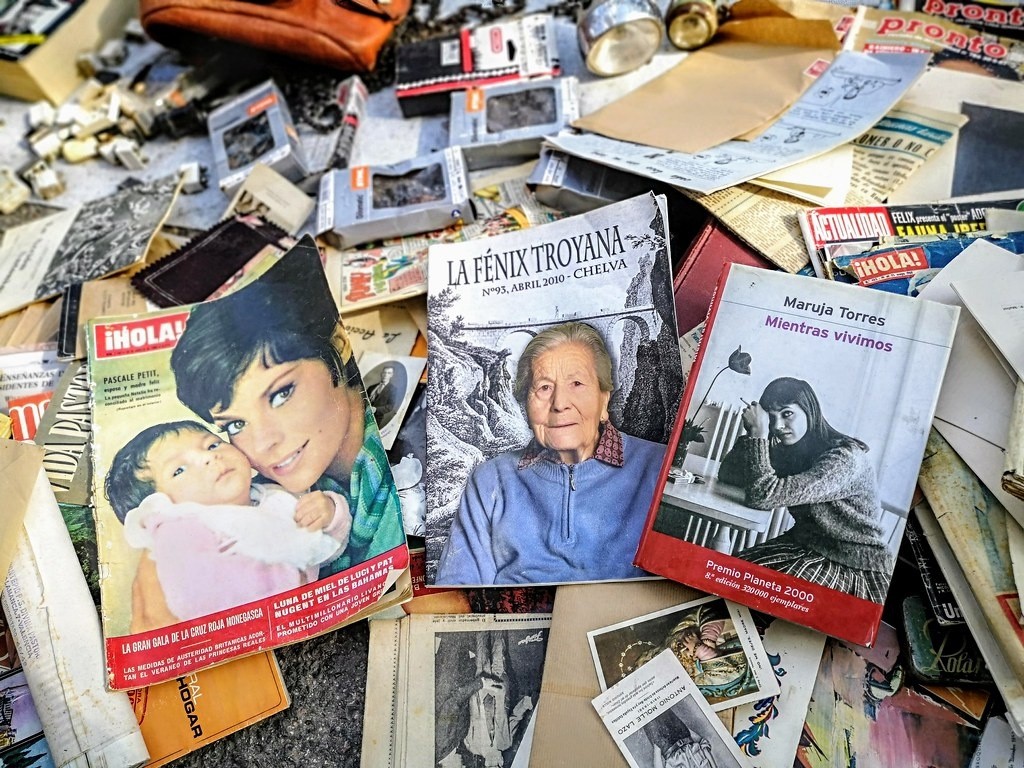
[
  {"xmin": 0, "ymin": 0, "xmax": 1024, "ymax": 768},
  {"xmin": 83, "ymin": 235, "xmax": 413, "ymax": 695},
  {"xmin": 425, "ymin": 192, "xmax": 686, "ymax": 588},
  {"xmin": 633, "ymin": 260, "xmax": 962, "ymax": 649}
]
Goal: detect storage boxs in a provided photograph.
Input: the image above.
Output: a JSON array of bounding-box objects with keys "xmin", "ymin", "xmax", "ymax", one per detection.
[
  {"xmin": 209, "ymin": 18, "xmax": 580, "ymax": 245},
  {"xmin": 1, "ymin": 0, "xmax": 139, "ymax": 104}
]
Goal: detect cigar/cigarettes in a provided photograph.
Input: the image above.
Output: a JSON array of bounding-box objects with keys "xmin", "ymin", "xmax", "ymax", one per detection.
[{"xmin": 740, "ymin": 397, "xmax": 751, "ymax": 406}]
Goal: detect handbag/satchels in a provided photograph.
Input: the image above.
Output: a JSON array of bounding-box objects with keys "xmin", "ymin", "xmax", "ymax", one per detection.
[{"xmin": 139, "ymin": 0, "xmax": 410, "ymax": 72}]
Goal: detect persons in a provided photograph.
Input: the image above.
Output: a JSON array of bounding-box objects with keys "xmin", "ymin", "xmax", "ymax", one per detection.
[
  {"xmin": 434, "ymin": 319, "xmax": 672, "ymax": 584},
  {"xmin": 718, "ymin": 376, "xmax": 894, "ymax": 606},
  {"xmin": 362, "ymin": 363, "xmax": 401, "ymax": 431},
  {"xmin": 123, "ymin": 280, "xmax": 405, "ymax": 636},
  {"xmin": 462, "ymin": 630, "xmax": 535, "ymax": 768},
  {"xmin": 633, "ymin": 602, "xmax": 759, "ymax": 698},
  {"xmin": 105, "ymin": 418, "xmax": 349, "ymax": 620}
]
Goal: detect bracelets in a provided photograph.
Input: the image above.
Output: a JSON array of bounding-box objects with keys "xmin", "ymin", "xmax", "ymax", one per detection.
[
  {"xmin": 692, "ymin": 642, "xmax": 704, "ymax": 656},
  {"xmin": 701, "ymin": 638, "xmax": 717, "ymax": 643}
]
